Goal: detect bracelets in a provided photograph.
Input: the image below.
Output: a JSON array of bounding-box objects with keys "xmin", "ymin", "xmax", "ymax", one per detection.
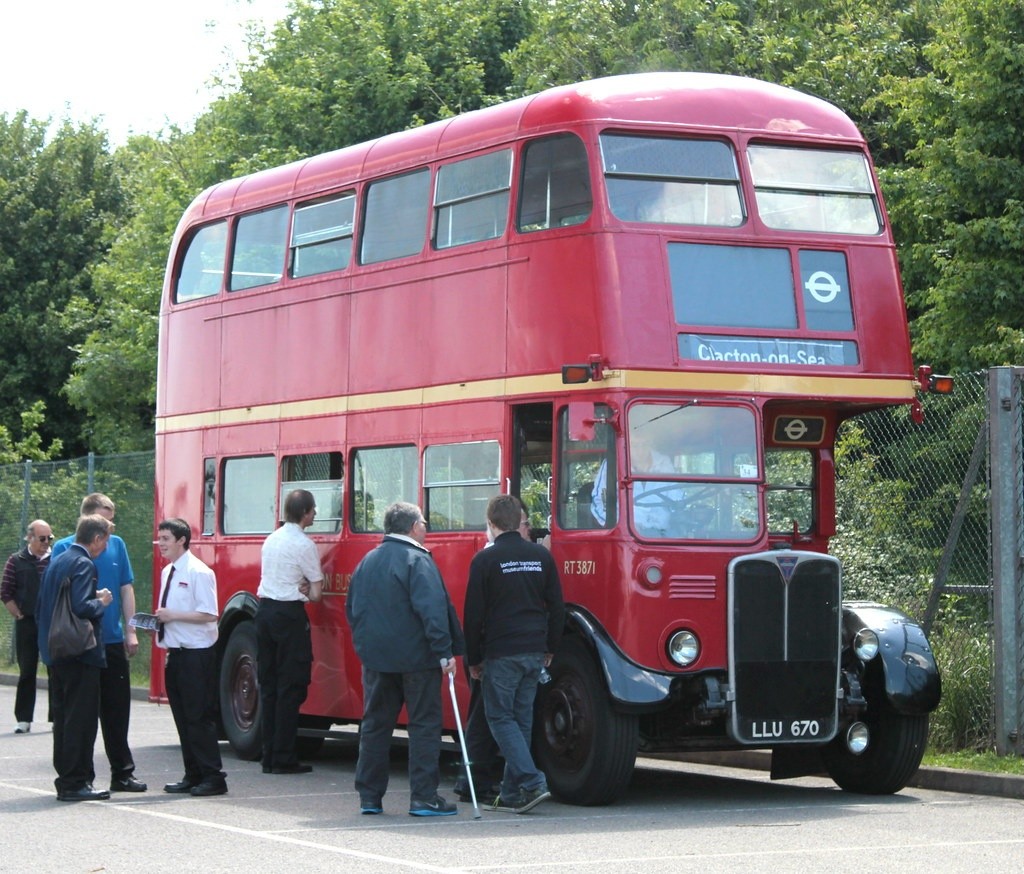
[{"xmin": 15, "ymin": 613, "xmax": 22, "ymax": 619}]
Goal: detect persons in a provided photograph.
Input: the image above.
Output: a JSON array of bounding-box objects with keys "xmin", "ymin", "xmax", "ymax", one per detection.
[
  {"xmin": 38, "ymin": 492, "xmax": 148, "ymax": 800},
  {"xmin": 345, "ymin": 501, "xmax": 465, "ymax": 816},
  {"xmin": 0, "ymin": 519, "xmax": 54, "ymax": 734},
  {"xmin": 256, "ymin": 489, "xmax": 324, "ymax": 773},
  {"xmin": 154, "ymin": 518, "xmax": 228, "ymax": 796},
  {"xmin": 453, "ymin": 493, "xmax": 563, "ymax": 813},
  {"xmin": 590, "ymin": 420, "xmax": 684, "ymax": 532}
]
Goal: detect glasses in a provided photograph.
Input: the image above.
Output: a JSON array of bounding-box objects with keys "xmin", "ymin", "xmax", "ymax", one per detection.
[
  {"xmin": 518, "ymin": 520, "xmax": 530, "ymax": 529},
  {"xmin": 39, "ymin": 536, "xmax": 53, "ymax": 542}
]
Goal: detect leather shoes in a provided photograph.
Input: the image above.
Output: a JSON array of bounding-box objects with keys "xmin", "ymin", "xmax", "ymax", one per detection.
[
  {"xmin": 191, "ymin": 778, "xmax": 229, "ymax": 797},
  {"xmin": 163, "ymin": 774, "xmax": 198, "ymax": 793}
]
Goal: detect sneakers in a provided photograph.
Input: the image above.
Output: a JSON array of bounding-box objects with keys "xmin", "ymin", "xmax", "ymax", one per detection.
[
  {"xmin": 481, "ymin": 796, "xmax": 526, "ymax": 813},
  {"xmin": 14, "ymin": 722, "xmax": 31, "ymax": 733},
  {"xmin": 109, "ymin": 773, "xmax": 147, "ymax": 791},
  {"xmin": 56, "ymin": 780, "xmax": 110, "ymax": 802},
  {"xmin": 359, "ymin": 796, "xmax": 384, "ymax": 814},
  {"xmin": 409, "ymin": 789, "xmax": 458, "ymax": 817},
  {"xmin": 514, "ymin": 783, "xmax": 551, "ymax": 814}
]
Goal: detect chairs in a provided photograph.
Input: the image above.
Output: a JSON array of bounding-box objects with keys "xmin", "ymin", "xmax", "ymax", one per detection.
[{"xmin": 577, "ymin": 481, "xmax": 603, "ymax": 529}]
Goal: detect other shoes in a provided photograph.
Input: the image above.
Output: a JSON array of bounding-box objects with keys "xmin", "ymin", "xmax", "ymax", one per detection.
[{"xmin": 260, "ymin": 755, "xmax": 314, "ymax": 775}]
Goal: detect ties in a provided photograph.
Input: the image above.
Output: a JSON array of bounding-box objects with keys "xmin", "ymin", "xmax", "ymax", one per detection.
[{"xmin": 157, "ymin": 566, "xmax": 175, "ymax": 642}]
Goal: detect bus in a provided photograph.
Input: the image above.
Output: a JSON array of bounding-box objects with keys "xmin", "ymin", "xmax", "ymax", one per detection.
[{"xmin": 149, "ymin": 70, "xmax": 953, "ymax": 806}]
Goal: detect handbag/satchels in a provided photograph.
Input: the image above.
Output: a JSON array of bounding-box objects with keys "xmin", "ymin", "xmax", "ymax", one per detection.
[{"xmin": 48, "ymin": 578, "xmax": 98, "ymax": 663}]
